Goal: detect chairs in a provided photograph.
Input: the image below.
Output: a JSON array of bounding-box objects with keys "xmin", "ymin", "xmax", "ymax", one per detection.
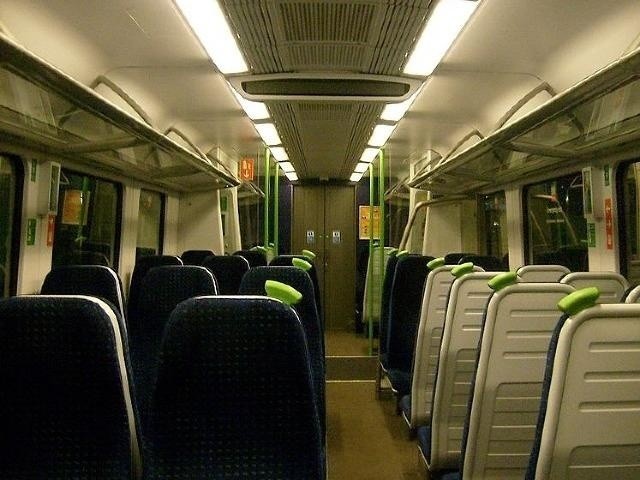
[
  {"xmin": 1, "ymin": 242, "xmax": 330, "ymax": 480},
  {"xmin": 357, "ymin": 242, "xmax": 639, "ymax": 480}
]
